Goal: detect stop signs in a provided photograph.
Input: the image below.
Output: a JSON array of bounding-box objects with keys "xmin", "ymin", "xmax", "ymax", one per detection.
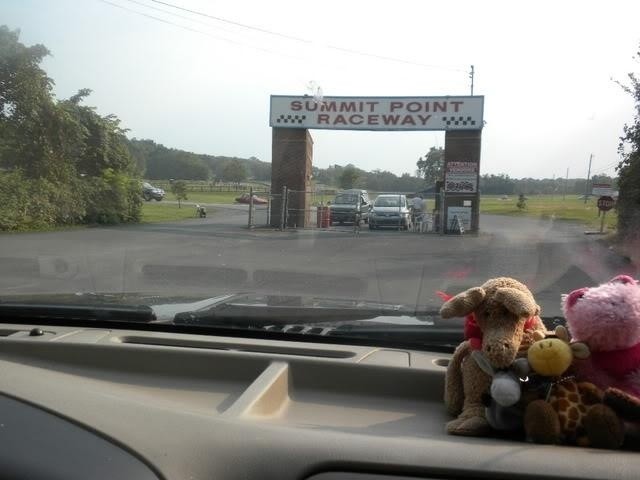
[{"xmin": 598, "ymin": 196, "xmax": 614, "ymax": 211}]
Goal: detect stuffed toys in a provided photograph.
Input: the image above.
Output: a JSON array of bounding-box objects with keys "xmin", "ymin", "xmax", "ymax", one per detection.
[{"xmin": 433, "ymin": 270, "xmax": 639, "ymax": 452}]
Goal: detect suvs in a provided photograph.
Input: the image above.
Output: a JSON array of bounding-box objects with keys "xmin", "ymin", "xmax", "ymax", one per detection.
[
  {"xmin": 139, "ymin": 182, "xmax": 166, "ymax": 201},
  {"xmin": 328, "ymin": 188, "xmax": 372, "ymax": 226}
]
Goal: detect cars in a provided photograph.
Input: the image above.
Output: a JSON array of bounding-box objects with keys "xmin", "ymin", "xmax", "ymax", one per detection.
[
  {"xmin": 236, "ymin": 193, "xmax": 268, "ymax": 205},
  {"xmin": 367, "ymin": 194, "xmax": 413, "ymax": 230}
]
